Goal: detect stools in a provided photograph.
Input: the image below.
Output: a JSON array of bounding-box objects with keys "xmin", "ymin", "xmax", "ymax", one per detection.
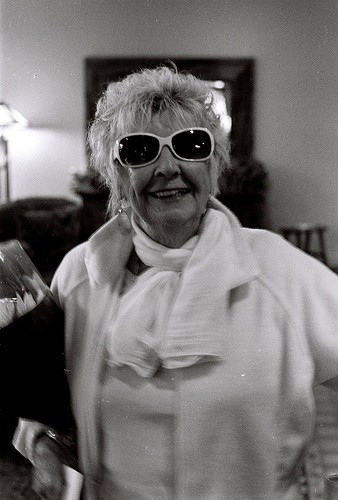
[{"xmin": 281, "ymin": 222, "xmax": 327, "ymax": 264}]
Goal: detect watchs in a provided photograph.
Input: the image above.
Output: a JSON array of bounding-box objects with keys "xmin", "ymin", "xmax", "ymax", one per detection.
[{"xmin": 44, "ymin": 427, "xmax": 76, "ymax": 453}]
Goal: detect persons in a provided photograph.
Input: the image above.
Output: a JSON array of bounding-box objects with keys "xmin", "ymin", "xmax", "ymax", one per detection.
[{"xmin": 12, "ymin": 68, "xmax": 338, "ymax": 499}]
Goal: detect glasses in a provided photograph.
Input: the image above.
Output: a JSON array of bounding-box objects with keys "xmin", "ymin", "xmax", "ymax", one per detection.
[{"xmin": 111, "ymin": 128, "xmax": 215, "ymax": 167}]
